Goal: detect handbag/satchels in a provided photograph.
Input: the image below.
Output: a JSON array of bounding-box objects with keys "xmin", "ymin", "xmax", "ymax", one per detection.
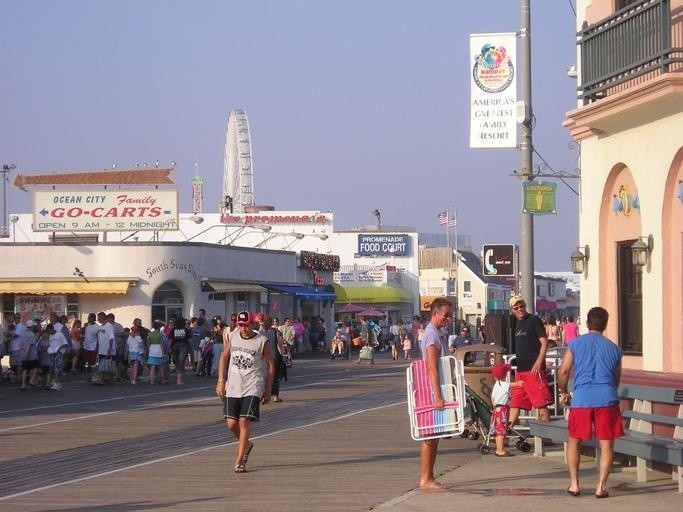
[
  {"xmin": 98, "ymin": 359, "xmax": 118, "ymax": 377},
  {"xmin": 359, "ymin": 345, "xmax": 374, "ymax": 361}
]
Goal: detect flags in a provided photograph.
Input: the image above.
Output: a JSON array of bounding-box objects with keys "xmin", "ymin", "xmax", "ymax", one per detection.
[
  {"xmin": 437, "ymin": 210, "xmax": 447, "ymax": 226},
  {"xmin": 448, "ymin": 216, "xmax": 456, "ymax": 228}
]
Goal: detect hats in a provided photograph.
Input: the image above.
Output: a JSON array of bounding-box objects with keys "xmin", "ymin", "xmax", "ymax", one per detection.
[
  {"xmin": 509, "ymin": 296, "xmax": 525, "ymax": 308},
  {"xmin": 234, "ymin": 310, "xmax": 255, "ymax": 325},
  {"xmin": 492, "ymin": 363, "xmax": 512, "ymax": 379},
  {"xmin": 25, "ymin": 319, "xmax": 38, "ymax": 328}
]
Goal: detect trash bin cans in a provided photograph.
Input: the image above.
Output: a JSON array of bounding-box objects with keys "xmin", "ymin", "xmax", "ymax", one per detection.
[{"xmin": 456, "ymin": 344, "xmax": 504, "ymax": 407}]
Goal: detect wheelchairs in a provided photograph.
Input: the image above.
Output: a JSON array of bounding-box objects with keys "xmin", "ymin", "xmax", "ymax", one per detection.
[{"xmin": 329, "ymin": 336, "xmax": 350, "ymax": 360}]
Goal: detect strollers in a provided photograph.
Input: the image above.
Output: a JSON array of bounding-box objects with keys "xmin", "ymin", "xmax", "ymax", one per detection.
[{"xmin": 450, "ymin": 379, "xmax": 531, "ymax": 455}]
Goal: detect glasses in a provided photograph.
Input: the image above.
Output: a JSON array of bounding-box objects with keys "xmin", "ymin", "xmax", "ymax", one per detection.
[
  {"xmin": 437, "ymin": 311, "xmax": 453, "ymax": 318},
  {"xmin": 511, "ymin": 305, "xmax": 524, "ymax": 311}
]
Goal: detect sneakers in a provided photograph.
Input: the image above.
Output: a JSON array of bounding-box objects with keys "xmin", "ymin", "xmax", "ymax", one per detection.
[{"xmin": 49, "ymin": 381, "xmax": 63, "ymax": 392}]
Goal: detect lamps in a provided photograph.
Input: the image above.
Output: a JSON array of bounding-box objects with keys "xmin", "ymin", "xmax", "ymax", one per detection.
[
  {"xmin": 570, "ymin": 245, "xmax": 590, "ymax": 274},
  {"xmin": 631, "ymin": 235, "xmax": 654, "ymax": 273}
]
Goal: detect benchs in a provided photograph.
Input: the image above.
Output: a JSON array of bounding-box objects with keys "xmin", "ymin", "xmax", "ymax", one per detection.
[
  {"xmin": 528, "ymin": 381, "xmax": 639, "ymax": 470},
  {"xmin": 602, "ymin": 385, "xmax": 683, "ymax": 493}
]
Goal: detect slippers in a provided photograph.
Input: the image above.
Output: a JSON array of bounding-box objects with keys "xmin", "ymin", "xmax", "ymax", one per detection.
[
  {"xmin": 234, "ymin": 463, "xmax": 246, "ymax": 473},
  {"xmin": 494, "ymin": 449, "xmax": 515, "ymax": 457},
  {"xmin": 240, "ymin": 442, "xmax": 253, "ymax": 465},
  {"xmin": 566, "ymin": 486, "xmax": 580, "ymax": 497},
  {"xmin": 595, "ymin": 489, "xmax": 609, "ymax": 498},
  {"xmin": 419, "ymin": 479, "xmax": 450, "ymax": 491}
]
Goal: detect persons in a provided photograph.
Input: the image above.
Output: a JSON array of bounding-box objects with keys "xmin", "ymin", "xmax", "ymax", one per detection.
[
  {"xmin": 558, "ymin": 307, "xmax": 625, "ymax": 498},
  {"xmin": 489, "ymin": 362, "xmax": 524, "ymax": 456},
  {"xmin": 419, "ymin": 298, "xmax": 453, "ymax": 491},
  {"xmin": 508, "ymin": 295, "xmax": 554, "ymax": 445},
  {"xmin": 2, "ymin": 309, "xmax": 580, "ymax": 403},
  {"xmin": 216, "ymin": 312, "xmax": 275, "ymax": 473}
]
